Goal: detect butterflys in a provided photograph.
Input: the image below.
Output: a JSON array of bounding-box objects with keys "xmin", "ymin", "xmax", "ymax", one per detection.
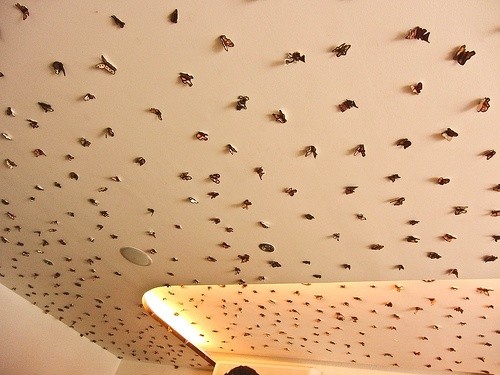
[{"xmin": 0, "ymin": 2, "xmax": 500, "ymax": 374}]
